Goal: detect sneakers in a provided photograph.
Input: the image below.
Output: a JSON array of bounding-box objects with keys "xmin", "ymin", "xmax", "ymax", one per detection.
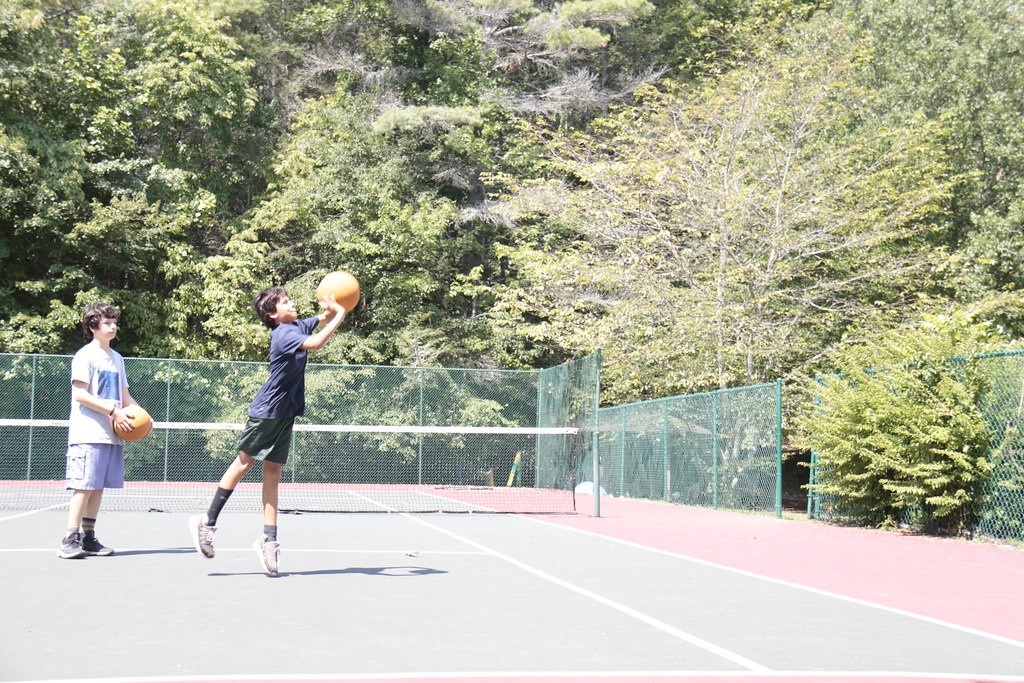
[
  {"xmin": 82, "ymin": 536, "xmax": 113, "ymax": 556},
  {"xmin": 261, "ymin": 540, "xmax": 281, "ymax": 576},
  {"xmin": 190, "ymin": 515, "xmax": 216, "ymax": 559},
  {"xmin": 57, "ymin": 537, "xmax": 86, "ymax": 560}
]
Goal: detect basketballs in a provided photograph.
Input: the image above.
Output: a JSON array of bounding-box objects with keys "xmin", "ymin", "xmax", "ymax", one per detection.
[
  {"xmin": 317, "ymin": 270, "xmax": 360, "ymax": 311},
  {"xmin": 113, "ymin": 405, "xmax": 150, "ymax": 440}
]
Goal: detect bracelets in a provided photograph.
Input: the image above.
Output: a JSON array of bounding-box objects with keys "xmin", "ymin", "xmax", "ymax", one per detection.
[{"xmin": 109, "ymin": 407, "xmax": 116, "ymax": 417}]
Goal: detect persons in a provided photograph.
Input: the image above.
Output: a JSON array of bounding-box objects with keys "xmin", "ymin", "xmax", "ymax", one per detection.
[
  {"xmin": 192, "ymin": 287, "xmax": 345, "ymax": 576},
  {"xmin": 58, "ymin": 303, "xmax": 153, "ymax": 558}
]
